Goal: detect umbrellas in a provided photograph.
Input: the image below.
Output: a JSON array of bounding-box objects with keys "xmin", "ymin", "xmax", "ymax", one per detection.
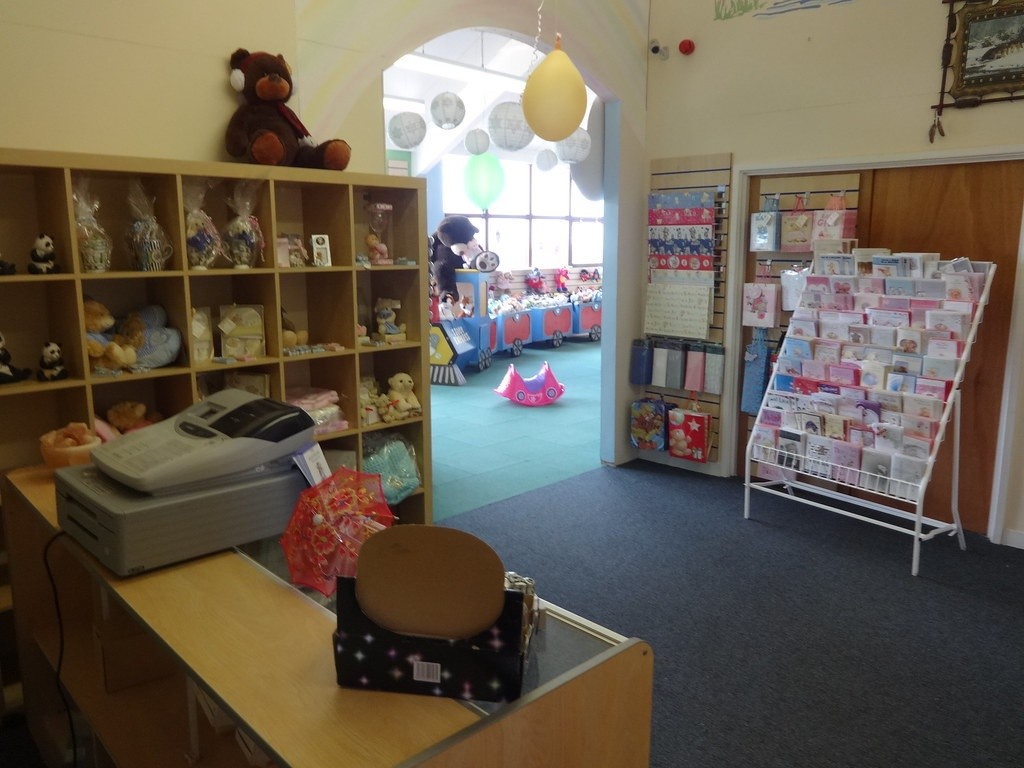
[{"xmin": 285, "ymin": 466, "xmax": 399, "ymax": 597}]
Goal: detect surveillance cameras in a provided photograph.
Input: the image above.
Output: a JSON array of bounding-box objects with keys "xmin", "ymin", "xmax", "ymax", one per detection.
[{"xmin": 650, "ymin": 39, "xmax": 660, "ymax": 54}]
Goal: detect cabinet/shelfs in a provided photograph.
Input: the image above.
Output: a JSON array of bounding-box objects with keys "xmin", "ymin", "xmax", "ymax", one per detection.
[
  {"xmin": 0, "ymin": 472, "xmax": 655, "ymax": 767},
  {"xmin": 0, "ymin": 146, "xmax": 434, "ymax": 611},
  {"xmin": 745, "ymin": 252, "xmax": 996, "ymax": 576}
]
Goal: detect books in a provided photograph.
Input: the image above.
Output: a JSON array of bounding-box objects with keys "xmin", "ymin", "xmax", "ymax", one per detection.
[{"xmin": 752, "ymin": 238, "xmax": 993, "ymax": 502}]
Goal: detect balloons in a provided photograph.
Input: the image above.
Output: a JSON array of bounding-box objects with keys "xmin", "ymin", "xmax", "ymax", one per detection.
[
  {"xmin": 522, "ymin": 50, "xmax": 587, "ymax": 141},
  {"xmin": 464, "ymin": 154, "xmax": 506, "ymax": 211}
]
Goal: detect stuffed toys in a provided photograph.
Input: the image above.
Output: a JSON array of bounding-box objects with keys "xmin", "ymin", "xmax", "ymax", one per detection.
[
  {"xmin": 373, "ymin": 373, "xmax": 421, "ymax": 422},
  {"xmin": 429, "ymin": 215, "xmax": 499, "ymax": 306},
  {"xmin": 375, "ymin": 298, "xmax": 406, "ymax": 334},
  {"xmin": 225, "ymin": 48, "xmax": 351, "ymax": 170},
  {"xmin": 0, "ymin": 215, "xmax": 308, "ymax": 434},
  {"xmin": 365, "ymin": 234, "xmax": 388, "ymax": 260},
  {"xmin": 439, "ymin": 266, "xmax": 602, "ymax": 320}
]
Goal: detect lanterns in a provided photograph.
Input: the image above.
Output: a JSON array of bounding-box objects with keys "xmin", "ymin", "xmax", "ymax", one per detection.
[{"xmin": 389, "ymin": 89, "xmax": 591, "ymax": 172}]
